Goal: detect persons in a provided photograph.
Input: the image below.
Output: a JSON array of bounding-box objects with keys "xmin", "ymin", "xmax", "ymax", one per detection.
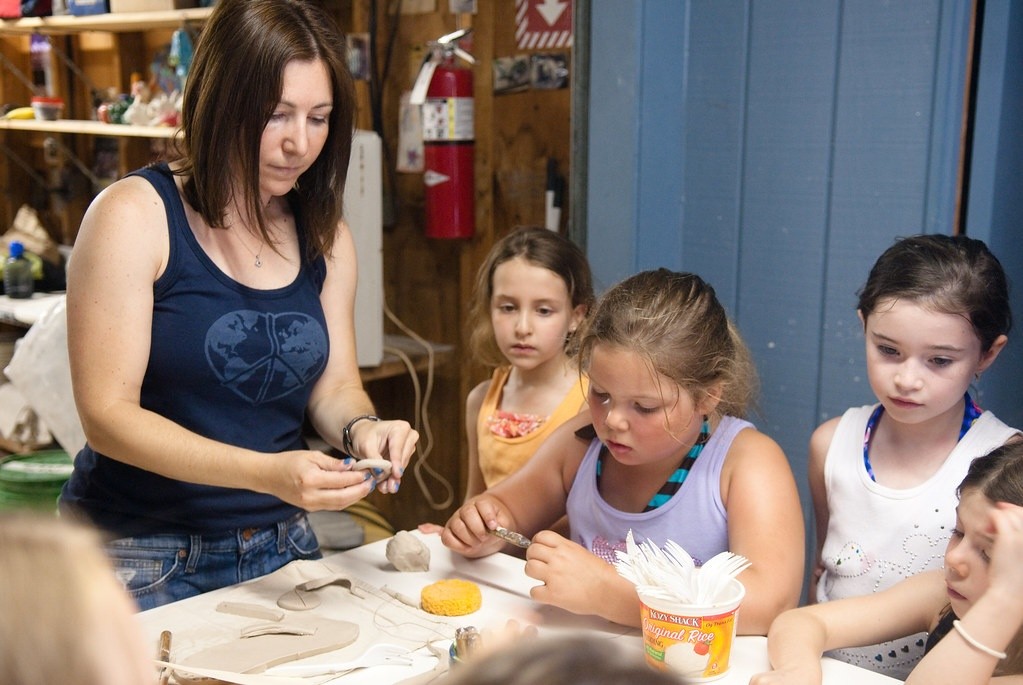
[
  {"xmin": 55, "ymin": 1, "xmax": 421, "ymax": 612},
  {"xmin": 0, "ymin": 510, "xmax": 157, "ymax": 685},
  {"xmin": 417, "ymin": 225, "xmax": 597, "ymax": 535},
  {"xmin": 808, "ymin": 233, "xmax": 1023, "ymax": 681},
  {"xmin": 748, "ymin": 432, "xmax": 1023, "ymax": 685},
  {"xmin": 439, "ymin": 266, "xmax": 809, "ymax": 636},
  {"xmin": 448, "ymin": 631, "xmax": 684, "ymax": 685}
]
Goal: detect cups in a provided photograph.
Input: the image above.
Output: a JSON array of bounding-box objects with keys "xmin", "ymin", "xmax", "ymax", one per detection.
[{"xmin": 30, "ymin": 97, "xmax": 64, "ymax": 120}]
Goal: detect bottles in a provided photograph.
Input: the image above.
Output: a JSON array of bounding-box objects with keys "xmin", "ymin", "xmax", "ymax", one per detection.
[
  {"xmin": 2, "ymin": 242, "xmax": 33, "ymax": 298},
  {"xmin": 30, "ymin": 35, "xmax": 55, "ymax": 96}
]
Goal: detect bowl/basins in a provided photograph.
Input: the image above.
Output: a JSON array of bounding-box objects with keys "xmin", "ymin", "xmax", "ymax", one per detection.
[{"xmin": 638, "ymin": 578, "xmax": 747, "ymax": 684}]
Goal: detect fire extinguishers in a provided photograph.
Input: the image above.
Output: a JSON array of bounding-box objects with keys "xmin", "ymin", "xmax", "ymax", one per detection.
[{"xmin": 408, "ymin": 28, "xmax": 479, "ymax": 240}]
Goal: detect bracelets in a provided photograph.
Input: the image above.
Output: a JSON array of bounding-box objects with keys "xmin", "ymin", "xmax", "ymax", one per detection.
[
  {"xmin": 952, "ymin": 620, "xmax": 1007, "ymax": 660},
  {"xmin": 342, "ymin": 415, "xmax": 381, "ymax": 460}
]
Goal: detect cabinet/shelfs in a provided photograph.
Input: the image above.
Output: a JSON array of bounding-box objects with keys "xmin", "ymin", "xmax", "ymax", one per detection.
[{"xmin": 0, "ymin": 10, "xmax": 456, "ymax": 383}]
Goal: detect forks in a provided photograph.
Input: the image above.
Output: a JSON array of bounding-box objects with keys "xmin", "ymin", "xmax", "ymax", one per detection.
[{"xmin": 610, "ymin": 528, "xmax": 753, "ymax": 604}]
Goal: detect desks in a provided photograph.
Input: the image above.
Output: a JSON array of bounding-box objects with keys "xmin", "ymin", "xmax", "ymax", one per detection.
[{"xmin": 135, "ymin": 528, "xmax": 908, "ymax": 685}]
